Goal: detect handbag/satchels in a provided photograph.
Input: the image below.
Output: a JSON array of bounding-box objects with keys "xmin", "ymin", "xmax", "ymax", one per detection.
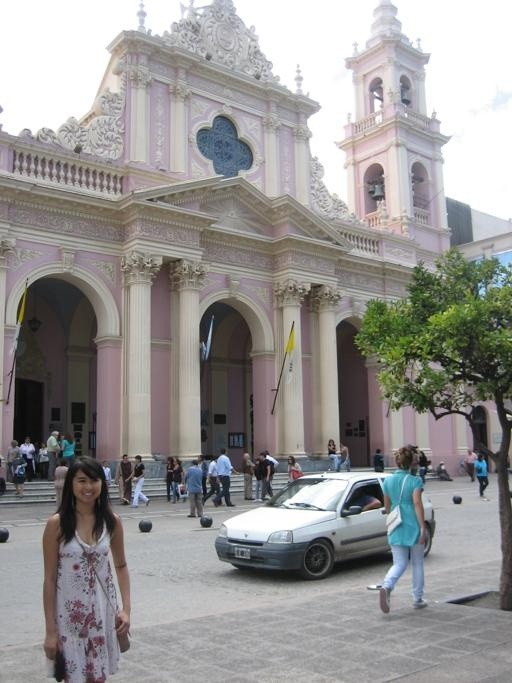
[
  {"xmin": 293, "ymin": 469, "xmax": 304, "ymax": 480},
  {"xmin": 386, "ymin": 505, "xmax": 403, "ymax": 535},
  {"xmin": 115, "ymin": 604, "xmax": 132, "ymax": 652}
]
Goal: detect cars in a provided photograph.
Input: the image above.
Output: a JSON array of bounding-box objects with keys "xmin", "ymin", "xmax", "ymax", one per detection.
[{"xmin": 214, "ymin": 467, "xmax": 435, "ymax": 581}]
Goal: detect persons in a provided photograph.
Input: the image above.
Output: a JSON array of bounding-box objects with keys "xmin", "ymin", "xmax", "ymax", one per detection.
[
  {"xmin": 373, "ymin": 448, "xmax": 384, "ymax": 472},
  {"xmin": 378, "ymin": 447, "xmax": 430, "ymax": 614},
  {"xmin": 411, "ymin": 449, "xmax": 488, "ymax": 497},
  {"xmin": 5, "ymin": 431, "xmax": 75, "ymax": 510},
  {"xmin": 241, "ymin": 450, "xmax": 281, "ymax": 503},
  {"xmin": 166, "ymin": 448, "xmax": 241, "ymax": 517},
  {"xmin": 328, "ymin": 439, "xmax": 350, "ymax": 473},
  {"xmin": 345, "ymin": 486, "xmax": 381, "ymax": 512},
  {"xmin": 286, "ymin": 456, "xmax": 302, "ymax": 500},
  {"xmin": 43, "ymin": 456, "xmax": 132, "ymax": 682},
  {"xmin": 102, "ymin": 454, "xmax": 150, "ymax": 508}
]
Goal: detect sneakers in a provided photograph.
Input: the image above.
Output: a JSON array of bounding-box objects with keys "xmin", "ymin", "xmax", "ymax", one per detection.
[
  {"xmin": 412, "ymin": 598, "xmax": 427, "ymax": 608},
  {"xmin": 380, "ymin": 585, "xmax": 390, "ymax": 613}
]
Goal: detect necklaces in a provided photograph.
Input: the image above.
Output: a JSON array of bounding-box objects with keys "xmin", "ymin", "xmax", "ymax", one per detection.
[{"xmin": 81, "ymin": 527, "xmax": 91, "ymax": 540}]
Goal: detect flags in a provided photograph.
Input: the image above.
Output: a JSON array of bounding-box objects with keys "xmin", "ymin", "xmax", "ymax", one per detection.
[
  {"xmin": 285, "ymin": 326, "xmax": 298, "ymax": 385},
  {"xmin": 199, "ymin": 314, "xmax": 215, "ymax": 359},
  {"xmin": 7, "ymin": 283, "xmax": 27, "ymax": 357}
]
